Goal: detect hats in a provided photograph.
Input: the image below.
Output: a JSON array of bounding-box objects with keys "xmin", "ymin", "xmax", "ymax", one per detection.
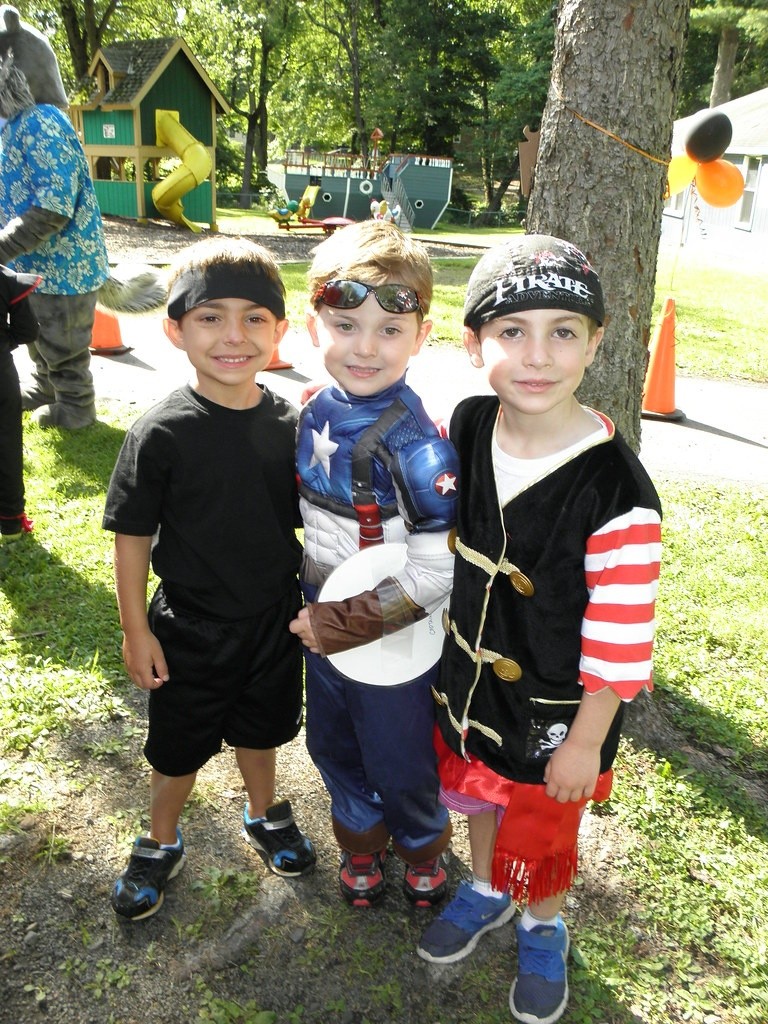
[{"xmin": 464, "ymin": 235, "xmax": 605, "ymax": 327}]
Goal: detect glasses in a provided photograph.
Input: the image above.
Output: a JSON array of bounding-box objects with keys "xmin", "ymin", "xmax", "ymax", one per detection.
[{"xmin": 313, "ymin": 279, "xmax": 428, "ymax": 314}]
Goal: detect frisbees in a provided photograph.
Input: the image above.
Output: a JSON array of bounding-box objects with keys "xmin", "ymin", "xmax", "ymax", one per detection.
[{"xmin": 318, "ymin": 542, "xmax": 450, "ymax": 688}]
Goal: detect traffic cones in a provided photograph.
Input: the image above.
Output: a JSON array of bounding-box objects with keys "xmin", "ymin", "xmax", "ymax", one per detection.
[
  {"xmin": 89, "ymin": 298, "xmax": 132, "ymax": 355},
  {"xmin": 262, "ymin": 346, "xmax": 293, "ymax": 370},
  {"xmin": 641, "ymin": 299, "xmax": 686, "ymax": 423}
]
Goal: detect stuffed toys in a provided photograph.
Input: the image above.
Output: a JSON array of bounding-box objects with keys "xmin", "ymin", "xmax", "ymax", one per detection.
[{"xmin": 0, "ymin": 7, "xmax": 173, "ymax": 431}]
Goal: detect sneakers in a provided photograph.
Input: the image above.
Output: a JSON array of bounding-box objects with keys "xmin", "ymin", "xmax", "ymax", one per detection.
[
  {"xmin": 416, "ymin": 880, "xmax": 516, "ymax": 964},
  {"xmin": 242, "ymin": 799, "xmax": 317, "ymax": 877},
  {"xmin": 508, "ymin": 913, "xmax": 570, "ymax": 1024},
  {"xmin": 110, "ymin": 827, "xmax": 187, "ymax": 921},
  {"xmin": 339, "ymin": 847, "xmax": 387, "ymax": 908},
  {"xmin": 403, "ymin": 841, "xmax": 453, "ymax": 907},
  {"xmin": 0, "ymin": 511, "xmax": 24, "ymax": 540}
]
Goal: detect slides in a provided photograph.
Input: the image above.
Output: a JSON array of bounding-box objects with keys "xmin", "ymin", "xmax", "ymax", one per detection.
[
  {"xmin": 294, "ymin": 185, "xmax": 319, "ymax": 223},
  {"xmin": 151, "ymin": 113, "xmax": 212, "ymax": 234}
]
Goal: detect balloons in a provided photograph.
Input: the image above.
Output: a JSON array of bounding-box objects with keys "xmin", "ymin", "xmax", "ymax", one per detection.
[
  {"xmin": 665, "ymin": 156, "xmax": 698, "ymax": 196},
  {"xmin": 696, "ymin": 160, "xmax": 745, "ymax": 208},
  {"xmin": 686, "ymin": 112, "xmax": 733, "ymax": 165}
]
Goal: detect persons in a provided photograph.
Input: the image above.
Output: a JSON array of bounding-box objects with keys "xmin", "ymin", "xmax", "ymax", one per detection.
[
  {"xmin": 109, "ymin": 236, "xmax": 324, "ymax": 921},
  {"xmin": 0, "ymin": 271, "xmax": 40, "ymax": 542},
  {"xmin": 290, "ymin": 218, "xmax": 464, "ymax": 920},
  {"xmin": 415, "ymin": 234, "xmax": 664, "ymax": 1024}
]
